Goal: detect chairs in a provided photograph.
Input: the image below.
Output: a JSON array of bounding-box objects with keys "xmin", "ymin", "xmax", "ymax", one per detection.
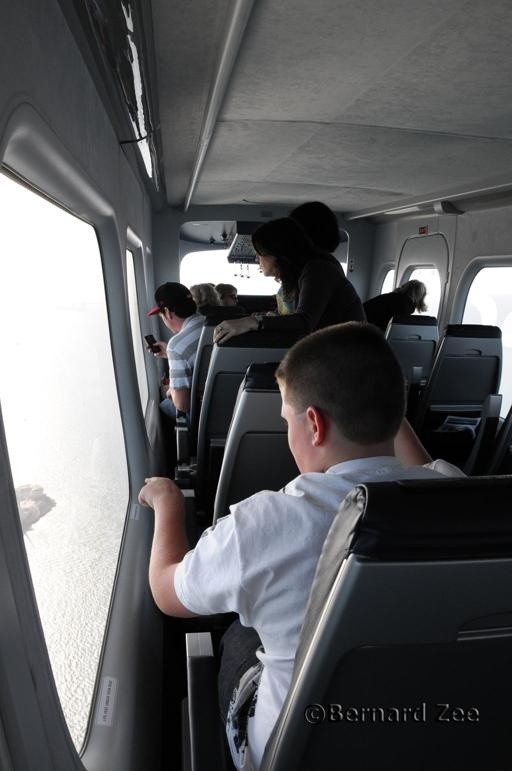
[{"xmin": 168, "ymin": 303, "xmax": 512, "ymax": 771}]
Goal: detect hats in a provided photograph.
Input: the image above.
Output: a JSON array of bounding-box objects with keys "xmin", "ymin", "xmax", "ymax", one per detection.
[{"xmin": 146, "ymin": 282, "xmax": 192, "ymax": 316}]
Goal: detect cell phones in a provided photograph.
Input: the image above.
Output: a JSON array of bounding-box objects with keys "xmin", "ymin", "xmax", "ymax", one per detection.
[{"xmin": 144, "ymin": 334, "xmax": 162, "ymax": 353}]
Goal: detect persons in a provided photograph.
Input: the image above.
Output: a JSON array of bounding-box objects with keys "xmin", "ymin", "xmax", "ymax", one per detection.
[
  {"xmin": 138, "ymin": 320, "xmax": 449, "ymax": 771},
  {"xmin": 144, "ymin": 201, "xmax": 363, "ymax": 427},
  {"xmin": 363, "ymin": 279, "xmax": 428, "ymax": 326}
]
